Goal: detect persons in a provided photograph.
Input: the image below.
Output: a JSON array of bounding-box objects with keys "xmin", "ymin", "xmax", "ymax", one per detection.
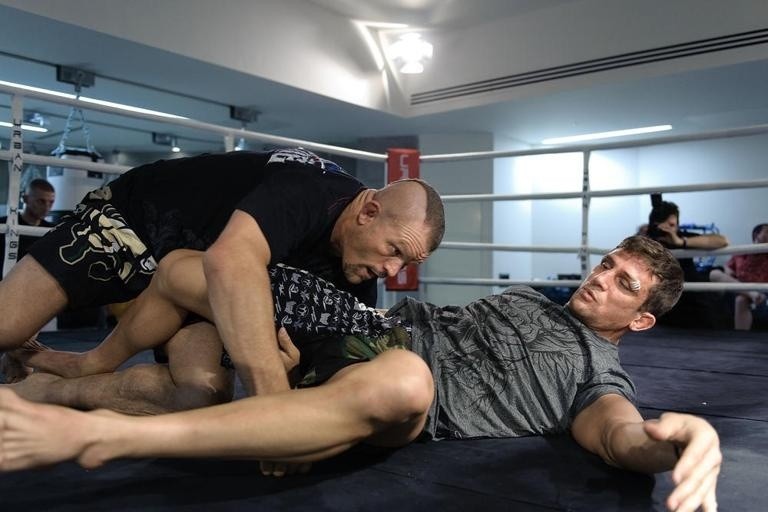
[
  {"xmin": 709, "ymin": 224, "xmax": 768, "ymax": 330},
  {"xmin": 635, "ymin": 203, "xmax": 726, "ymax": 285},
  {"xmin": 1, "ymin": 148, "xmax": 445, "ymax": 477},
  {"xmin": 1, "ymin": 178, "xmax": 58, "ymax": 258},
  {"xmin": 0, "ymin": 236, "xmax": 723, "ymax": 511}
]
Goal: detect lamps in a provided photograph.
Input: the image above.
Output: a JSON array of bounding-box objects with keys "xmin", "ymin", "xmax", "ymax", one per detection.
[
  {"xmin": 170, "ymin": 137, "xmax": 181, "ymax": 152},
  {"xmin": 0, "ymin": 121, "xmax": 49, "ymax": 134}
]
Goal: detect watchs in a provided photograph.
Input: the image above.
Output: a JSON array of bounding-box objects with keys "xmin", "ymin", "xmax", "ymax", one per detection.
[{"xmin": 682, "ymin": 235, "xmax": 688, "ymax": 249}]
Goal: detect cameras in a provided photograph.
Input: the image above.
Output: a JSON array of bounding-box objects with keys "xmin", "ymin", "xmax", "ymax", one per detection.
[{"xmin": 647, "ymin": 192, "xmax": 672, "ymax": 249}]
